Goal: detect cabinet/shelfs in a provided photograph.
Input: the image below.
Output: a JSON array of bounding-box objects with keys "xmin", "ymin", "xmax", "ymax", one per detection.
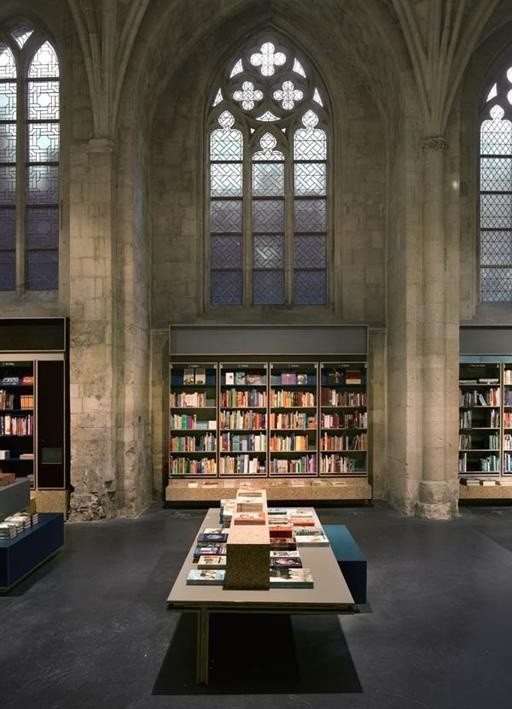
[
  {"xmin": 458, "ymin": 356, "xmax": 512, "ymax": 500},
  {"xmin": 0, "ymin": 353, "xmax": 69, "ymax": 521},
  {"xmin": 165, "ymin": 361, "xmax": 371, "ymax": 502}
]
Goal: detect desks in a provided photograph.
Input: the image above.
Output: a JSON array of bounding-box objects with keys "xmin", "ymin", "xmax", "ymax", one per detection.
[{"xmin": 166, "ymin": 507, "xmax": 355, "ymax": 685}]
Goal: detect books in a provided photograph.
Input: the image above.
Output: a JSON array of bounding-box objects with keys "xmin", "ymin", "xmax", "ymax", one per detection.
[
  {"xmin": 188, "ymin": 479, "xmax": 348, "ymax": 487},
  {"xmin": 321, "ymin": 368, "xmax": 368, "ymax": 473},
  {"xmin": 187, "ymin": 499, "xmax": 235, "ymax": 584},
  {"xmin": 0, "ymin": 367, "xmax": 38, "ymax": 540},
  {"xmin": 168, "ymin": 367, "xmax": 217, "ymax": 474},
  {"xmin": 270, "ymin": 369, "xmax": 317, "ymax": 472},
  {"xmin": 234, "ymin": 488, "xmax": 266, "ymax": 525},
  {"xmin": 459, "ymin": 369, "xmax": 512, "ymax": 486},
  {"xmin": 267, "ymin": 507, "xmax": 330, "ymax": 588},
  {"xmin": 219, "ymin": 371, "xmax": 266, "ymax": 474}
]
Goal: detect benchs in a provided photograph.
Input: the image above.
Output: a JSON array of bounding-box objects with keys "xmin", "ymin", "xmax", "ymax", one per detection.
[{"xmin": 321, "ymin": 524, "xmax": 368, "ymax": 604}]
